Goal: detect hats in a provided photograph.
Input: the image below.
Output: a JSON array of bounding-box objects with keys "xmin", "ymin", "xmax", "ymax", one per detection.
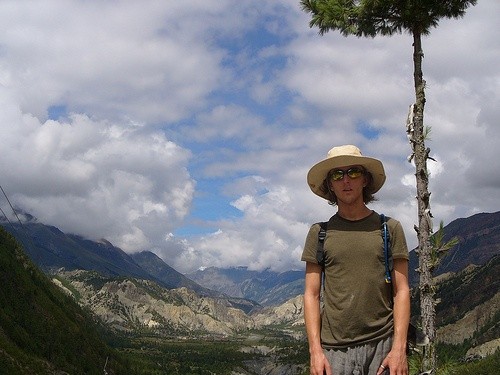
[{"xmin": 307, "ymin": 145, "xmax": 386, "ymax": 200}]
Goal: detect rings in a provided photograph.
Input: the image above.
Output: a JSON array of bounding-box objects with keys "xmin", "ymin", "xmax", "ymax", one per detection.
[{"xmin": 380, "ymin": 365, "xmax": 385, "ymax": 369}]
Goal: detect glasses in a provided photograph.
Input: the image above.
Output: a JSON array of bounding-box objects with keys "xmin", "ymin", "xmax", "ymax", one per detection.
[{"xmin": 330, "ymin": 167, "xmax": 365, "ymax": 181}]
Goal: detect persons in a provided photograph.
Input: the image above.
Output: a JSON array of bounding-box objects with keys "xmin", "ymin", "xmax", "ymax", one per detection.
[{"xmin": 301, "ymin": 144, "xmax": 410, "ymax": 375}]
